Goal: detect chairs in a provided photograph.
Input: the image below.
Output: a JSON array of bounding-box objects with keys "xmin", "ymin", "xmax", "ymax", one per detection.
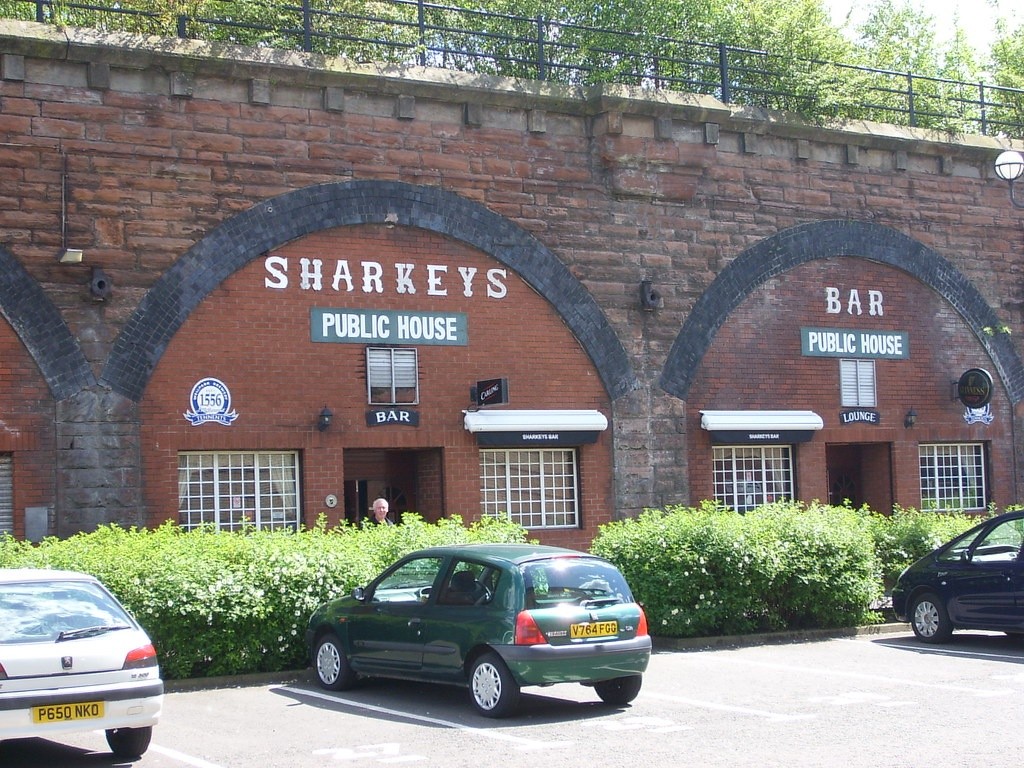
[{"xmin": 445, "ymin": 570, "xmax": 477, "ymax": 604}]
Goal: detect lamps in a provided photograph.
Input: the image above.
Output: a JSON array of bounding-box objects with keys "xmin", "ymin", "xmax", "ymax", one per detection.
[
  {"xmin": 56, "ymin": 248, "xmax": 83, "ymax": 264},
  {"xmin": 318, "ymin": 405, "xmax": 334, "ymax": 431},
  {"xmin": 903, "ymin": 406, "xmax": 918, "ymax": 429}
]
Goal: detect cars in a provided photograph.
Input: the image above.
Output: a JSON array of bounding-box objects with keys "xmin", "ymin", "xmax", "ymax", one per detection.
[
  {"xmin": 300, "ymin": 540, "xmax": 652, "ymax": 721},
  {"xmin": 892, "ymin": 508, "xmax": 1024, "ymax": 645},
  {"xmin": 0, "ymin": 565, "xmax": 164, "ymax": 763}
]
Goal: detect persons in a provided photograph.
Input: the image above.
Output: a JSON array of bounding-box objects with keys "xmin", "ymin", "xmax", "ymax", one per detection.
[{"xmin": 367, "ymin": 498, "xmax": 394, "ymax": 528}]
